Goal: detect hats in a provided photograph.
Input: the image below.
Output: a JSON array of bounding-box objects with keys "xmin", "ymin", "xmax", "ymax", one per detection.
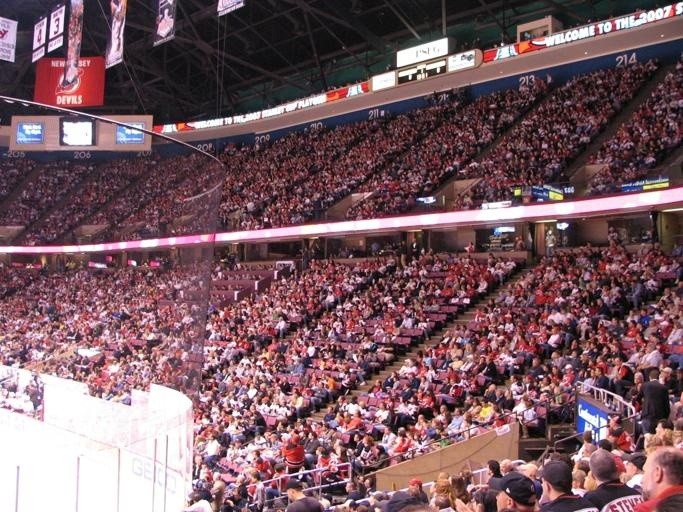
[
  {"xmin": 536, "ymin": 460, "xmax": 572, "ymax": 486},
  {"xmin": 408, "ymin": 478, "xmax": 423, "ymax": 486},
  {"xmin": 488, "ymin": 471, "xmax": 536, "ymax": 502},
  {"xmin": 622, "ymin": 451, "xmax": 647, "ymax": 469}
]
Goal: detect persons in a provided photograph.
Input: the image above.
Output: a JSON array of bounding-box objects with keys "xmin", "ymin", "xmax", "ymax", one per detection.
[{"xmin": 2, "ymin": 0, "xmax": 683, "ymax": 510}]
